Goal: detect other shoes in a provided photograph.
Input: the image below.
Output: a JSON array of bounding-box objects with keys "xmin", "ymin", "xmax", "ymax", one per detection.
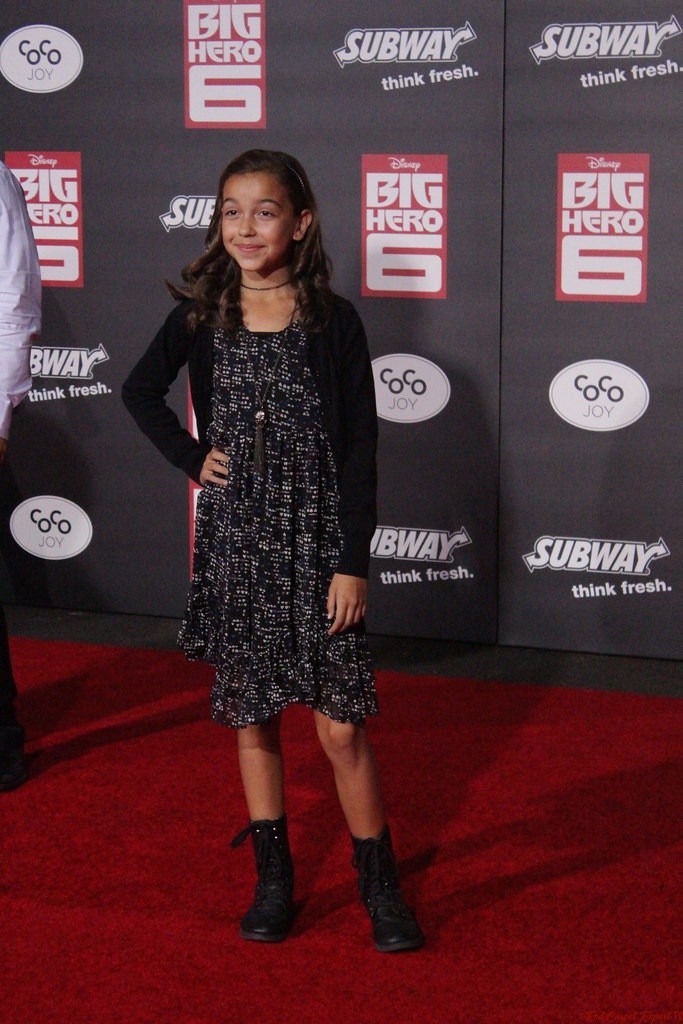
[{"xmin": 0, "ymin": 744, "xmax": 28, "ymax": 790}]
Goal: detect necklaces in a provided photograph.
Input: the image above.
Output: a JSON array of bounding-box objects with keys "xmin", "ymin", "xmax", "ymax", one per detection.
[
  {"xmin": 237, "ymin": 298, "xmax": 298, "ymax": 471},
  {"xmin": 238, "ymin": 279, "xmax": 290, "ymax": 291}
]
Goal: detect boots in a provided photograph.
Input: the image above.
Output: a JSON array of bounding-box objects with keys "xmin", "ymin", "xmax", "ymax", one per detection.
[
  {"xmin": 351, "ymin": 824, "xmax": 425, "ymax": 952},
  {"xmin": 231, "ymin": 812, "xmax": 293, "ymax": 941}
]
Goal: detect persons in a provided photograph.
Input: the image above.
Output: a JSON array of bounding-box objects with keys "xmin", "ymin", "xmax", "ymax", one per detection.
[
  {"xmin": 121, "ymin": 149, "xmax": 426, "ymax": 953},
  {"xmin": 0, "ymin": 160, "xmax": 41, "ymax": 791}
]
